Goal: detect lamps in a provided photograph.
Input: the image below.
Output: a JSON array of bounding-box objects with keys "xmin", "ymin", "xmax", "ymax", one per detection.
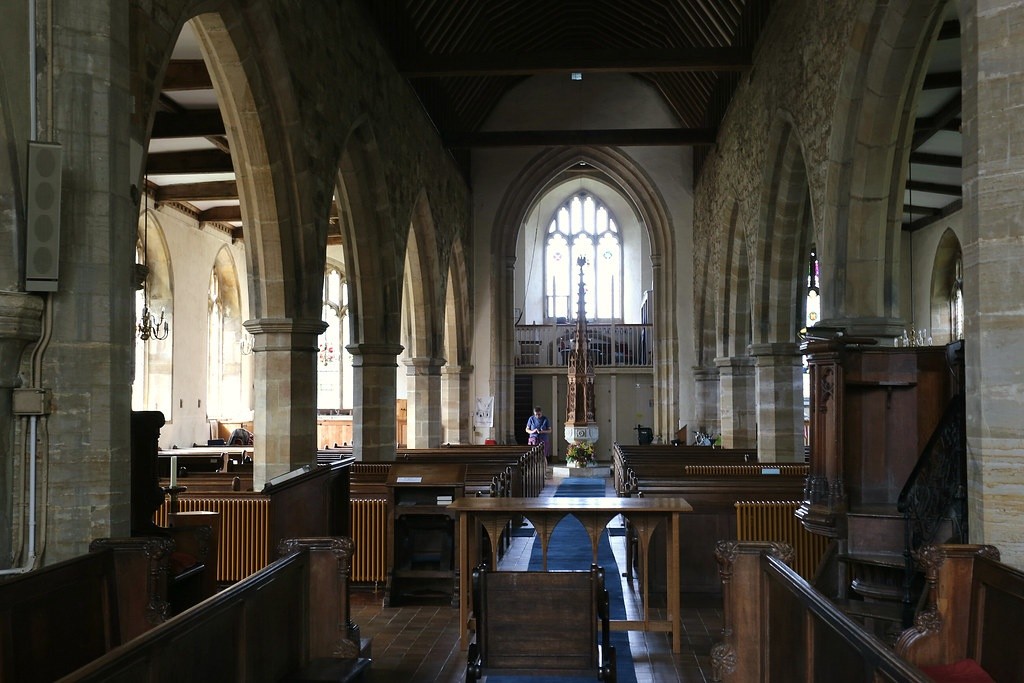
[
  {"xmin": 893, "ymin": 153, "xmax": 933, "ymax": 348},
  {"xmin": 136, "ymin": 170, "xmax": 169, "ymax": 341}
]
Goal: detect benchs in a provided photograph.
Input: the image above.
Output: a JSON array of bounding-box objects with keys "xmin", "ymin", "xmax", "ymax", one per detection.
[
  {"xmin": 316, "ymin": 442, "xmax": 547, "ymax": 567},
  {"xmin": 609, "ymin": 442, "xmax": 808, "ymax": 603}
]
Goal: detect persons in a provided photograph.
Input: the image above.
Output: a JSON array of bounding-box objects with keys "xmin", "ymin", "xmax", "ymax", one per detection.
[{"xmin": 525, "ymin": 406, "xmax": 552, "ymax": 479}]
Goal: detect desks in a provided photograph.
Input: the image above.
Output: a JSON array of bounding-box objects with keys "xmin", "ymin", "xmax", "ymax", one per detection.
[{"xmin": 448, "ymin": 495, "xmax": 695, "ymax": 656}]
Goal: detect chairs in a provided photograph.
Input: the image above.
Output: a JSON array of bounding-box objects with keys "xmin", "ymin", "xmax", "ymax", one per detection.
[{"xmin": 466, "ymin": 563, "xmax": 618, "ymax": 682}]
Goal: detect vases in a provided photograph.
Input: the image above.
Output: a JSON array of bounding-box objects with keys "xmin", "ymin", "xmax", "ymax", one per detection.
[{"xmin": 575, "ymin": 461, "xmax": 587, "ymax": 469}]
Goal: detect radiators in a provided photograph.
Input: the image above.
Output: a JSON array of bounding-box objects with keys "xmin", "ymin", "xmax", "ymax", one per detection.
[
  {"xmin": 685, "ymin": 464, "xmax": 810, "ymax": 476},
  {"xmin": 151, "ymin": 496, "xmax": 387, "ymax": 596},
  {"xmin": 733, "ymin": 500, "xmax": 832, "ymax": 581}
]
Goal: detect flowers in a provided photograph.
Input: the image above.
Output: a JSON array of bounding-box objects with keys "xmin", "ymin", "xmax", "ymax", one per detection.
[{"xmin": 564, "ymin": 442, "xmax": 600, "ymax": 468}]
